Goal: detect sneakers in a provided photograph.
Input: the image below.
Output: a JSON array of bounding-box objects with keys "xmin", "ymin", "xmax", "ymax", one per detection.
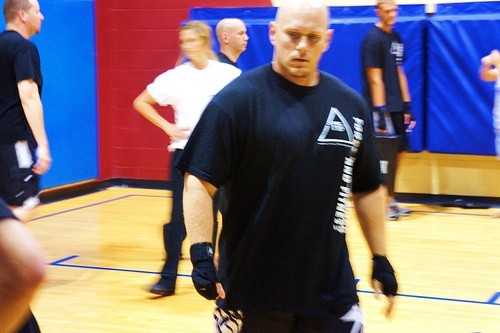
[
  {"xmin": 398, "ymin": 207, "xmax": 411, "ymax": 216},
  {"xmin": 386, "ymin": 199, "xmax": 398, "ymax": 220}
]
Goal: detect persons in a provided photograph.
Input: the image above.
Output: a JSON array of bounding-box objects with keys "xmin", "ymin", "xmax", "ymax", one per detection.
[
  {"xmin": 359, "ymin": 0, "xmax": 418, "ymax": 220},
  {"xmin": 215, "ymin": 16, "xmax": 250, "ymax": 75},
  {"xmin": 479, "ymin": 48, "xmax": 500, "ymax": 173},
  {"xmin": 0, "ymin": 197, "xmax": 49, "ymax": 332},
  {"xmin": 132, "ymin": 18, "xmax": 243, "ymax": 300},
  {"xmin": 0, "ymin": 0, "xmax": 51, "ymax": 229},
  {"xmin": 172, "ymin": 0, "xmax": 400, "ymax": 332}
]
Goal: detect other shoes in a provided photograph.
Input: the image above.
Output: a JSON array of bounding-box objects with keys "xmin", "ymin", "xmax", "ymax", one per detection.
[{"xmin": 149, "ymin": 277, "xmax": 176, "ymax": 295}]
